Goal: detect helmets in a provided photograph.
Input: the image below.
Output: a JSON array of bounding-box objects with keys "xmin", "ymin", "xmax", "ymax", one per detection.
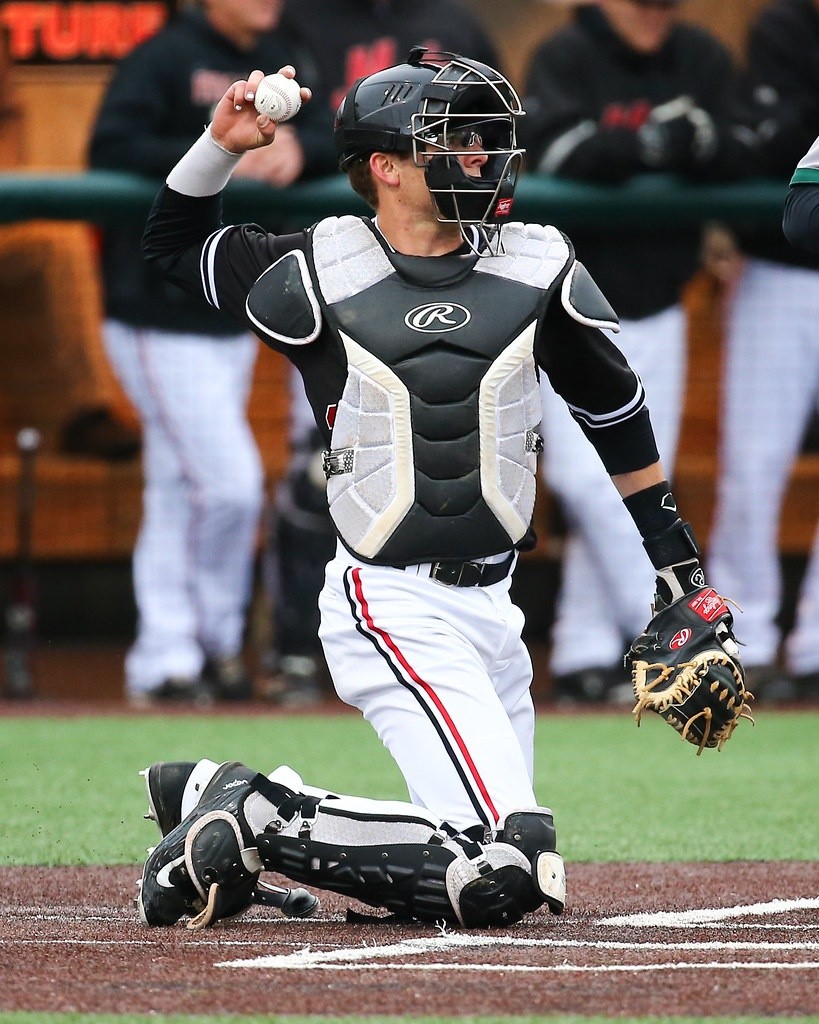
[{"xmin": 333, "ymin": 62, "xmax": 513, "ymax": 171}]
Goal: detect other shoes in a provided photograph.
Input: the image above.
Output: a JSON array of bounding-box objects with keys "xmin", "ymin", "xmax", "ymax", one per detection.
[
  {"xmin": 204, "ymin": 658, "xmax": 251, "ymax": 703},
  {"xmin": 126, "ymin": 684, "xmax": 210, "ymax": 712}
]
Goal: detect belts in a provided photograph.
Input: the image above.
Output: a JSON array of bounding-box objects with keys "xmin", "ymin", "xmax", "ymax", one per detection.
[{"xmin": 395, "ymin": 551, "xmax": 515, "ymax": 588}]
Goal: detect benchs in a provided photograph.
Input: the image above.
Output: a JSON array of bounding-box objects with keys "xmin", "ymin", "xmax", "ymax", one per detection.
[{"xmin": 0, "ymin": 60, "xmax": 819, "ymax": 659}]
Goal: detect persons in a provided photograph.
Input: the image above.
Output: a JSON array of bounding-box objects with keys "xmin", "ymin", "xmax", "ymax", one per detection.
[
  {"xmin": 84, "ymin": 0, "xmax": 819, "ymax": 714},
  {"xmin": 135, "ymin": 48, "xmax": 756, "ymax": 930}
]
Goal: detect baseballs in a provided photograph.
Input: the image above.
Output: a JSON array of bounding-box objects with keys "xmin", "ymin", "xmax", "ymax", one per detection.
[{"xmin": 253, "ymin": 73, "xmax": 303, "ymax": 124}]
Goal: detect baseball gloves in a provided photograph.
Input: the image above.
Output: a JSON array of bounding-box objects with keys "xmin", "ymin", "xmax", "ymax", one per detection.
[{"xmin": 629, "ymin": 584, "xmax": 747, "ymax": 749}]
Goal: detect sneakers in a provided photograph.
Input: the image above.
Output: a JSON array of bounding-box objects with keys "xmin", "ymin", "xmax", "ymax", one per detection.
[
  {"xmin": 139, "ymin": 764, "xmax": 192, "ymax": 839},
  {"xmin": 136, "ymin": 763, "xmax": 257, "ymax": 927}
]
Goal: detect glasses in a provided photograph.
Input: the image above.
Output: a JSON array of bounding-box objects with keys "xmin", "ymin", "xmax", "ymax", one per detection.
[{"xmin": 443, "ymin": 131, "xmax": 484, "ymax": 146}]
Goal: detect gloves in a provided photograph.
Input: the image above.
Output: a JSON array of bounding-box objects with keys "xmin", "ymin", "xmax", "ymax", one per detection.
[
  {"xmin": 620, "ymin": 109, "xmax": 674, "ymax": 167},
  {"xmin": 674, "ymin": 107, "xmax": 726, "ymax": 171}
]
